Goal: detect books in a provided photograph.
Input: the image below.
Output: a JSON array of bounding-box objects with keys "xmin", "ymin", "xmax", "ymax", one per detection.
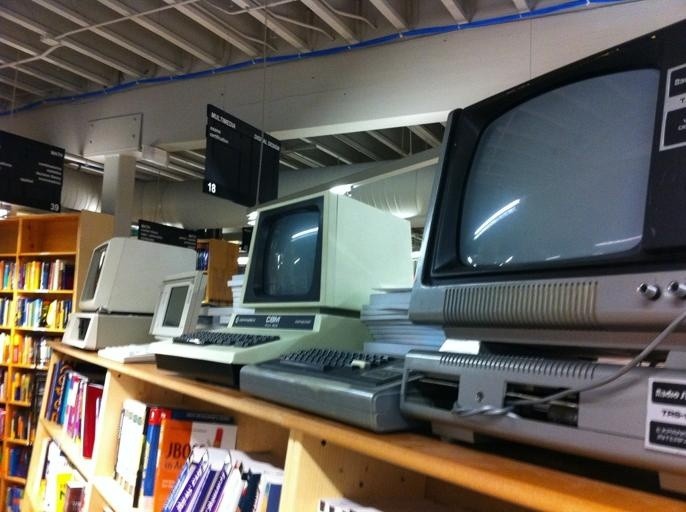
[
  {"xmin": 226, "ymin": 273, "xmax": 247, "ymax": 306},
  {"xmin": 76, "ymin": 367, "xmax": 283, "ymax": 511},
  {"xmin": 359, "ymin": 284, "xmax": 448, "ymax": 360},
  {"xmin": 1, "ymin": 255, "xmax": 76, "ymax": 511},
  {"xmin": 318, "ymin": 497, "xmax": 448, "ymax": 512}
]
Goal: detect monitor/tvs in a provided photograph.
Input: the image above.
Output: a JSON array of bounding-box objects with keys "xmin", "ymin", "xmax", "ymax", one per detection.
[
  {"xmin": 79, "ymin": 237, "xmax": 197, "ymax": 314},
  {"xmin": 148, "ymin": 270, "xmax": 206, "ymax": 341},
  {"xmin": 408, "ymin": 18, "xmax": 686, "ymax": 371},
  {"xmin": 239, "ymin": 190, "xmax": 413, "ymax": 312}
]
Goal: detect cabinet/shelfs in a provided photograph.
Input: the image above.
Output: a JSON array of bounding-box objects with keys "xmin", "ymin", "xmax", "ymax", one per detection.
[
  {"xmin": 19, "ymin": 340, "xmax": 685, "ymax": 512},
  {"xmin": 0, "ymin": 213, "xmax": 115, "ymax": 512}
]
